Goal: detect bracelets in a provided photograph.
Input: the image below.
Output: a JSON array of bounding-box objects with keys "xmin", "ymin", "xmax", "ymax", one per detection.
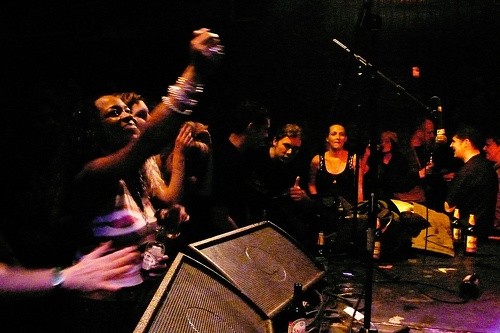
[
  {"xmin": 161, "ymin": 96, "xmax": 193, "ymax": 116},
  {"xmin": 162, "ymin": 76, "xmax": 205, "ymax": 94},
  {"xmin": 167, "ymin": 85, "xmax": 200, "ymax": 107},
  {"xmin": 53, "ymin": 266, "xmax": 64, "ymax": 289}
]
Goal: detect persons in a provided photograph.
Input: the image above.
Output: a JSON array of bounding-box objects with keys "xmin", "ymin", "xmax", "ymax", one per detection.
[
  {"xmin": 0, "ymin": 240, "xmax": 143, "ymax": 292},
  {"xmin": 482, "ymin": 131, "xmax": 500, "ymax": 241},
  {"xmin": 42, "ymin": 27, "xmax": 224, "ymax": 333},
  {"xmin": 444, "ymin": 127, "xmax": 499, "ymax": 293},
  {"xmin": 115, "ymin": 92, "xmax": 456, "ymax": 270}
]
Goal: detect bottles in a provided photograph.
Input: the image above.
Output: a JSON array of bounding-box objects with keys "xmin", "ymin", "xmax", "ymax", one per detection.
[
  {"xmin": 464, "ymin": 214, "xmax": 478, "ymax": 256},
  {"xmin": 451, "ymin": 207, "xmax": 462, "ymax": 241},
  {"xmin": 288, "ymin": 282, "xmax": 306, "ymax": 333},
  {"xmin": 316, "ymin": 232, "xmax": 325, "ymax": 265},
  {"xmin": 373, "ymin": 218, "xmax": 381, "ymax": 259}
]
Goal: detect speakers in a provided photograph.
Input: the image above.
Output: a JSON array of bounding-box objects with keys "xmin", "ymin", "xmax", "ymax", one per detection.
[
  {"xmin": 134, "ymin": 219, "xmax": 326, "ymax": 333},
  {"xmin": 333, "ymin": 217, "xmax": 412, "ymax": 262}
]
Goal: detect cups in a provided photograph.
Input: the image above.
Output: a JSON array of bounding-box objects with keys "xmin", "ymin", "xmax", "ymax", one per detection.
[
  {"xmin": 142, "ymin": 242, "xmax": 165, "ymax": 270},
  {"xmin": 437, "ymin": 128, "xmax": 446, "ymax": 141}
]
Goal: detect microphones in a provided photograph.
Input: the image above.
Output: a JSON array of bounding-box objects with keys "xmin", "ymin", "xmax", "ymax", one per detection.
[{"xmin": 436, "ymin": 101, "xmax": 447, "ymax": 144}]
